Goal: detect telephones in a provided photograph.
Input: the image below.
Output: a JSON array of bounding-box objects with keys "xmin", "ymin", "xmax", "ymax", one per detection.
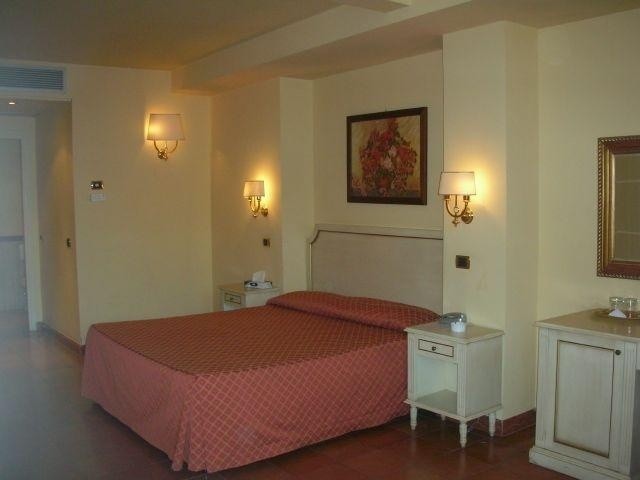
[{"xmin": 439, "ymin": 312, "xmax": 467, "ymax": 325}]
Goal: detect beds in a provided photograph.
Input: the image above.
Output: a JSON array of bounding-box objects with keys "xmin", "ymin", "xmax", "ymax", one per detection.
[{"xmin": 82, "ymin": 225, "xmax": 449, "ymax": 474}]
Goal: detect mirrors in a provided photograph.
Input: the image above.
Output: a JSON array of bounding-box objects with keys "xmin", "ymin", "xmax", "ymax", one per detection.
[{"xmin": 596, "ymin": 134, "xmax": 640, "ymax": 280}]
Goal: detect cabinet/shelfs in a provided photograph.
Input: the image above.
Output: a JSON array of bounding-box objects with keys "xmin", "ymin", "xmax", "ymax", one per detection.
[{"xmin": 528, "ymin": 305, "xmax": 640, "ymax": 480}]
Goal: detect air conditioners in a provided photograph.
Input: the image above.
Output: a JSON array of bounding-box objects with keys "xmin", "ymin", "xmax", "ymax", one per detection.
[{"xmin": 0, "ymin": 66, "xmax": 66, "ymax": 90}]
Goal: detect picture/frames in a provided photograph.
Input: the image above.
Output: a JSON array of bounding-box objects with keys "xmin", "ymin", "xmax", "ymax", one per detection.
[{"xmin": 345, "ymin": 105, "xmax": 427, "ymax": 207}]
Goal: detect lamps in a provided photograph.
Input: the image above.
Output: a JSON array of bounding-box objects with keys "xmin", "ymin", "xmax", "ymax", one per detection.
[
  {"xmin": 242, "ymin": 181, "xmax": 269, "ymax": 218},
  {"xmin": 147, "ymin": 110, "xmax": 186, "ymax": 159},
  {"xmin": 438, "ymin": 170, "xmax": 475, "ymax": 228}
]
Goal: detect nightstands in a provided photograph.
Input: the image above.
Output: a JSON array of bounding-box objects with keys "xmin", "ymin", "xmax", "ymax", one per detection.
[
  {"xmin": 403, "ymin": 315, "xmax": 505, "ymax": 450},
  {"xmin": 219, "ymin": 277, "xmax": 281, "ymax": 312}
]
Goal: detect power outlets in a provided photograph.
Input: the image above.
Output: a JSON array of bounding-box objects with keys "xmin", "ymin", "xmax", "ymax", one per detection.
[
  {"xmin": 456, "ymin": 255, "xmax": 470, "ymax": 270},
  {"xmin": 262, "ymin": 238, "xmax": 270, "ymax": 246}
]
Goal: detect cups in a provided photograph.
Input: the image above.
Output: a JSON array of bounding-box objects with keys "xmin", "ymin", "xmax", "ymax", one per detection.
[{"xmin": 609, "ymin": 296, "xmax": 640, "ymax": 316}]
